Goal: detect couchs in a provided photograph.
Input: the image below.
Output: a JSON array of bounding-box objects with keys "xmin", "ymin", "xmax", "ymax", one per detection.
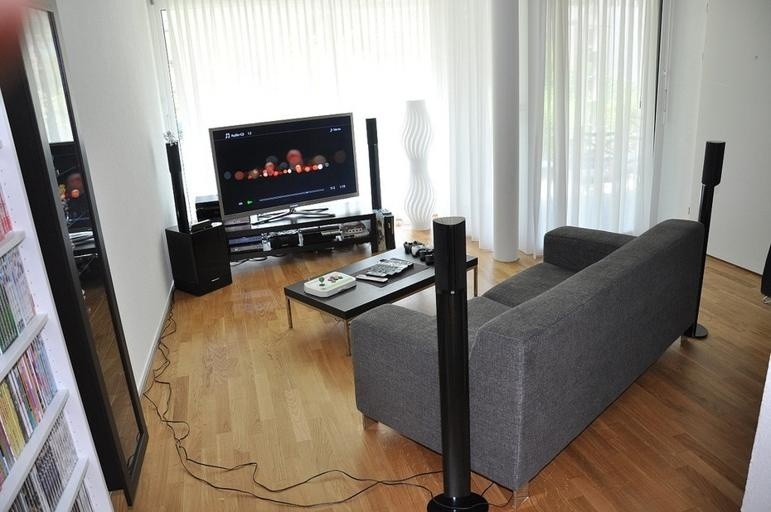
[{"xmin": 349, "ymin": 218, "xmax": 707, "ymax": 493}]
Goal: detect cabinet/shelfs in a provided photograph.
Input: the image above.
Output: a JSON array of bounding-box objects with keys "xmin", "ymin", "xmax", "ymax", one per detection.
[
  {"xmin": 227, "ymin": 204, "xmax": 379, "ymax": 272},
  {"xmin": 0, "ymin": 229, "xmax": 90, "ymax": 511}
]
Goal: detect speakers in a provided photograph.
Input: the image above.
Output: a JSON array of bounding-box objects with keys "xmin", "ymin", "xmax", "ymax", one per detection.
[
  {"xmin": 166, "ymin": 141, "xmax": 188, "ymax": 230},
  {"xmin": 427, "ymin": 216, "xmax": 489, "ymax": 511},
  {"xmin": 196, "ymin": 195, "xmax": 221, "ymax": 222},
  {"xmin": 685, "ymin": 140, "xmax": 726, "ymax": 339},
  {"xmin": 366, "ymin": 118, "xmax": 381, "ymax": 210},
  {"xmin": 165, "ymin": 223, "xmax": 232, "ymax": 296}
]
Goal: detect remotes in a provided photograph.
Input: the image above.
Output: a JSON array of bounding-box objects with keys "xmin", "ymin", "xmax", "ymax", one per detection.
[{"xmin": 356, "ymin": 258, "xmax": 414, "ymax": 284}]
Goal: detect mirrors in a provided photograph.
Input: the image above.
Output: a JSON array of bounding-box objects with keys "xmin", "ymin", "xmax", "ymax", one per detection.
[{"xmin": 11, "ymin": 1, "xmax": 150, "ymax": 508}]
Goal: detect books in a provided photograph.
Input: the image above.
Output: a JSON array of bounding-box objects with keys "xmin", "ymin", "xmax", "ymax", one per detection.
[{"xmin": 0, "ymin": 183, "xmax": 100, "ymax": 512}]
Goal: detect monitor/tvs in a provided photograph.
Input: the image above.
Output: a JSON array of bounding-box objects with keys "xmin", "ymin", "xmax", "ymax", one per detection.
[{"xmin": 209, "ymin": 113, "xmax": 359, "ymax": 225}]
